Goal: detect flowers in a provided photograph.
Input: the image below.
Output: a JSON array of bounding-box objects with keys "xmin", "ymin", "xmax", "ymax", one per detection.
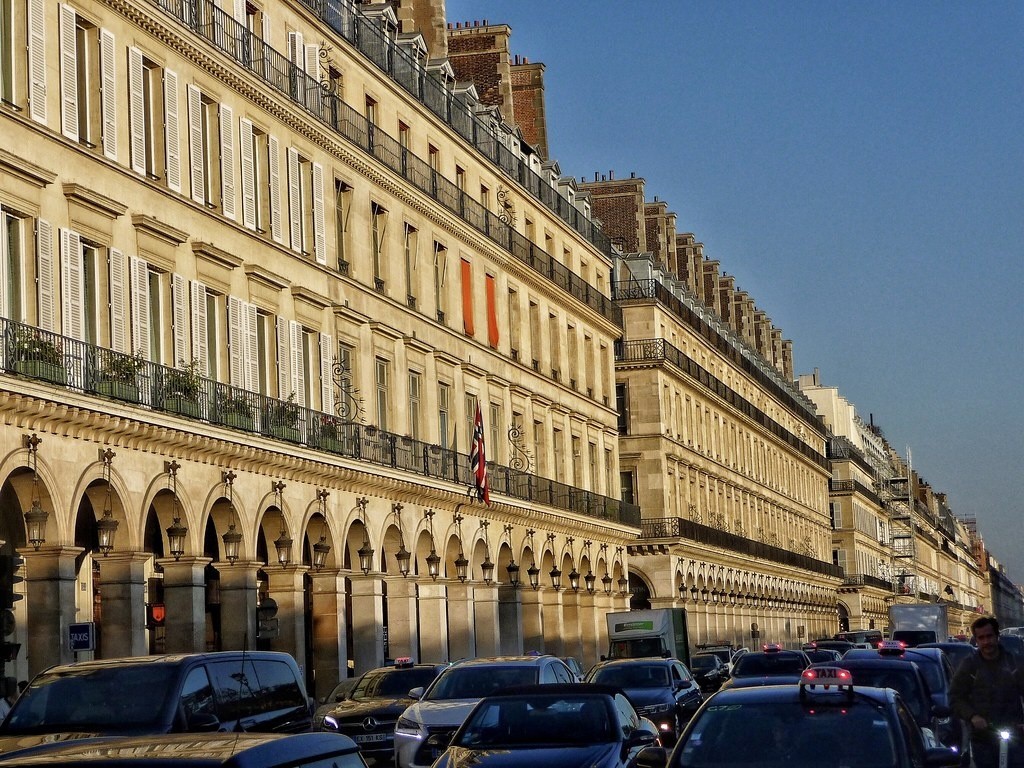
[
  {"xmin": 320, "ymin": 417, "xmax": 340, "ymax": 439},
  {"xmin": 12, "ymin": 329, "xmax": 63, "ymax": 365},
  {"xmin": 102, "ymin": 348, "xmax": 146, "ymax": 384},
  {"xmin": 161, "ymin": 356, "xmax": 202, "ymax": 400},
  {"xmin": 217, "ymin": 392, "xmax": 255, "ymax": 417},
  {"xmin": 269, "ymin": 391, "xmax": 300, "ymax": 428}
]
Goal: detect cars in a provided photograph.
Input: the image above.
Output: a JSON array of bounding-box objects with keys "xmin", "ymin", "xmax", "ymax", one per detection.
[
  {"xmin": 0, "ymin": 729, "xmax": 370, "ymax": 768},
  {"xmin": 313, "ymin": 629, "xmax": 1024, "ymax": 768}
]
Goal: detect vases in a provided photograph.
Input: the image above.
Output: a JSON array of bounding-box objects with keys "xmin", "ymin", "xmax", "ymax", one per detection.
[
  {"xmin": 220, "ymin": 412, "xmax": 255, "ymax": 431},
  {"xmin": 15, "ymin": 360, "xmax": 68, "ymax": 383},
  {"xmin": 269, "ymin": 426, "xmax": 301, "ymax": 441},
  {"xmin": 164, "ymin": 398, "xmax": 201, "ymax": 418},
  {"xmin": 310, "ymin": 435, "xmax": 341, "ymax": 452},
  {"xmin": 99, "ymin": 381, "xmax": 139, "ymax": 401}
]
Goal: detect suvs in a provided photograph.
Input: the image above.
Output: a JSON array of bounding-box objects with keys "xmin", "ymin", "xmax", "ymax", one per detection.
[{"xmin": 2, "ymin": 650, "xmax": 314, "ymax": 735}]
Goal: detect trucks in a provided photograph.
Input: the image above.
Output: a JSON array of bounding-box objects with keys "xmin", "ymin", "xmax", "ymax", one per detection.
[
  {"xmin": 888, "ymin": 602, "xmax": 949, "ymax": 647},
  {"xmin": 602, "ymin": 606, "xmax": 694, "ymax": 681}
]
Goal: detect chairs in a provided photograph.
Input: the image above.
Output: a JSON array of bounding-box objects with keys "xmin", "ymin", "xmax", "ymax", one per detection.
[
  {"xmin": 803, "ymin": 714, "xmax": 892, "ymax": 767},
  {"xmin": 715, "ymin": 707, "xmax": 778, "ymax": 768},
  {"xmin": 145, "ymin": 672, "xmax": 177, "ymax": 722},
  {"xmin": 75, "ymin": 676, "xmax": 116, "ymax": 723},
  {"xmin": 491, "ymin": 706, "xmax": 533, "ymax": 745}
]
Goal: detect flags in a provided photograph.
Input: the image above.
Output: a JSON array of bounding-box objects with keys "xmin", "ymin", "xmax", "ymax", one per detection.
[
  {"xmin": 470, "ymin": 399, "xmax": 493, "ymax": 508},
  {"xmin": 944, "ymin": 585, "xmax": 954, "ymax": 595}
]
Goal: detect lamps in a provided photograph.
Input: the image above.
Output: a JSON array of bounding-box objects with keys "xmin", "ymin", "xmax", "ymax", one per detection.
[
  {"xmin": 22, "ymin": 433, "xmax": 50, "ymax": 552},
  {"xmin": 164, "ymin": 460, "xmax": 188, "ymax": 562},
  {"xmin": 221, "ymin": 469, "xmax": 242, "ymax": 566},
  {"xmin": 313, "ymin": 488, "xmax": 628, "ymax": 598},
  {"xmin": 272, "ymin": 481, "xmax": 293, "ymax": 570},
  {"xmin": 678, "ymin": 558, "xmax": 839, "ymax": 617},
  {"xmin": 97, "ymin": 448, "xmax": 120, "ymax": 558},
  {"xmin": 861, "ymin": 594, "xmax": 889, "ymax": 621}
]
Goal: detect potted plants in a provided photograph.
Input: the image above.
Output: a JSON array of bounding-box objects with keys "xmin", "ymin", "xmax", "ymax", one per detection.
[
  {"xmin": 365, "ymin": 424, "xmax": 376, "ymax": 436},
  {"xmin": 402, "ymin": 434, "xmax": 414, "ymax": 445},
  {"xmin": 428, "ymin": 444, "xmax": 442, "ymax": 454}
]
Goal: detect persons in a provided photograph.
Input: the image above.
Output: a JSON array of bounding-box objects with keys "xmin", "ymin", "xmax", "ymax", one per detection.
[
  {"xmin": 949, "ymin": 617, "xmax": 1024, "ymax": 768},
  {"xmin": 948, "ymin": 636, "xmax": 954, "ymax": 643},
  {"xmin": 736, "ymin": 645, "xmax": 743, "ymax": 660},
  {"xmin": 0, "ymin": 677, "xmax": 27, "ymax": 727}
]
